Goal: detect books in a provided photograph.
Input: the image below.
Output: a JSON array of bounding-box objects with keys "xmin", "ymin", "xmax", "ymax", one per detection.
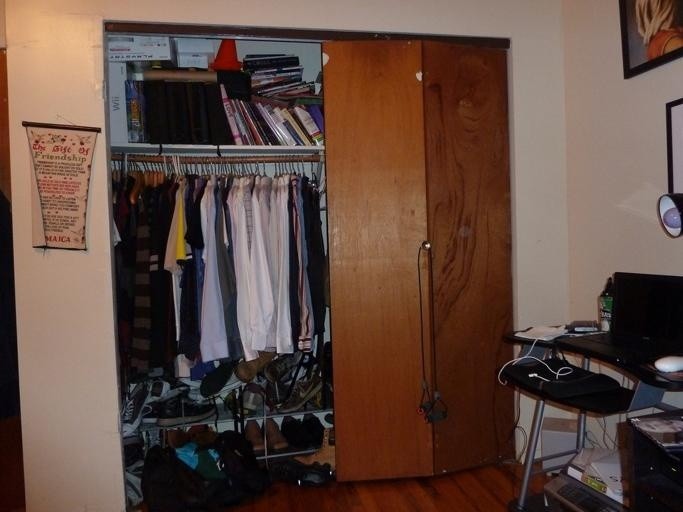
[
  {"xmin": 123, "ymin": 50, "xmax": 324, "ymax": 148},
  {"xmin": 571, "ymin": 320, "xmax": 599, "ymax": 333},
  {"xmin": 628, "ymin": 414, "xmax": 683, "ymax": 453}
]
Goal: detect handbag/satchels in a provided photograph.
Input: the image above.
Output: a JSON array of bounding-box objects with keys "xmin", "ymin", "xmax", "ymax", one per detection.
[{"xmin": 142, "ymin": 424, "xmax": 274, "ymax": 511}]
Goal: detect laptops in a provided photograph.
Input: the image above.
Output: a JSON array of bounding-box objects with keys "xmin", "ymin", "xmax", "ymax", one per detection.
[{"xmin": 557, "ymin": 272, "xmax": 683, "ymax": 365}]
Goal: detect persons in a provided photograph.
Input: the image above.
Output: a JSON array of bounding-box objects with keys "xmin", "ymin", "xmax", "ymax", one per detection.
[
  {"xmin": 633, "ymin": 0, "xmax": 682, "ymax": 62},
  {"xmin": 636, "ymin": 416, "xmax": 682, "ymax": 437}
]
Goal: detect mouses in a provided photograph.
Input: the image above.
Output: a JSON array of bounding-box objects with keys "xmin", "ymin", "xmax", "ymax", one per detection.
[{"xmin": 654, "ymin": 355, "xmax": 683, "ymax": 372}]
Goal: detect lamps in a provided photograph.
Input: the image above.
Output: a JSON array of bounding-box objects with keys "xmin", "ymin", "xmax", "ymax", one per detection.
[{"xmin": 656, "ymin": 193, "xmax": 683, "ymax": 237}]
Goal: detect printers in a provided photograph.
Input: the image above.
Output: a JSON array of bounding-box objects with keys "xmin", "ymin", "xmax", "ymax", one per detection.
[{"xmin": 542, "ymin": 447, "xmax": 625, "ymax": 511}]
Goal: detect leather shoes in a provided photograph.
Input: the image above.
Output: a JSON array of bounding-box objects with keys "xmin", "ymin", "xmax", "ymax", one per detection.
[{"xmin": 244, "ymin": 418, "xmax": 288, "ymax": 452}]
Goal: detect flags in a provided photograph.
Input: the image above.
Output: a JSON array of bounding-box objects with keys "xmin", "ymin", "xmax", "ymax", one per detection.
[{"xmin": 20, "ymin": 120, "xmax": 101, "ymax": 252}]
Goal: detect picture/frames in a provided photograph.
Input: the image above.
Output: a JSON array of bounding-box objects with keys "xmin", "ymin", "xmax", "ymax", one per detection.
[{"xmin": 618, "ymin": 1, "xmax": 682, "ymax": 194}]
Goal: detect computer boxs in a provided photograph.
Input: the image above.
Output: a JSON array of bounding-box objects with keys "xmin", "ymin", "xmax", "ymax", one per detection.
[{"xmin": 627, "ymin": 408, "xmax": 683, "ymax": 512}]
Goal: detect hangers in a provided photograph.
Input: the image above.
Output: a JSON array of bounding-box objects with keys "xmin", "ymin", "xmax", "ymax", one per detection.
[{"xmin": 106, "ymin": 150, "xmax": 326, "ymax": 211}]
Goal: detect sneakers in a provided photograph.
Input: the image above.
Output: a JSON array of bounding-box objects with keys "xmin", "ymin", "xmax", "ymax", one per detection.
[
  {"xmin": 120, "ymin": 344, "xmax": 331, "ymax": 439},
  {"xmin": 282, "ymin": 414, "xmax": 333, "ymax": 450},
  {"xmin": 303, "ymin": 461, "xmax": 332, "ymax": 487}
]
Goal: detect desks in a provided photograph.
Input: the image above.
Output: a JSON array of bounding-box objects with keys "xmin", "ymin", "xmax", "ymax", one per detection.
[{"xmin": 497, "ymin": 317, "xmax": 683, "ymax": 508}]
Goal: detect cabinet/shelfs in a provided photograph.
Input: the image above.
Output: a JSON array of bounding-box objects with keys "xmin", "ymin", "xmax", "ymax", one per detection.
[
  {"xmin": 118, "ymin": 406, "xmax": 334, "ymax": 463},
  {"xmin": 102, "ymin": 20, "xmax": 515, "ymax": 510}
]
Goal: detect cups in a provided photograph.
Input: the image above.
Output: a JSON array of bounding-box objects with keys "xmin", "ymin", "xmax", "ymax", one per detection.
[{"xmin": 599, "ymin": 290, "xmax": 614, "ymax": 332}]
